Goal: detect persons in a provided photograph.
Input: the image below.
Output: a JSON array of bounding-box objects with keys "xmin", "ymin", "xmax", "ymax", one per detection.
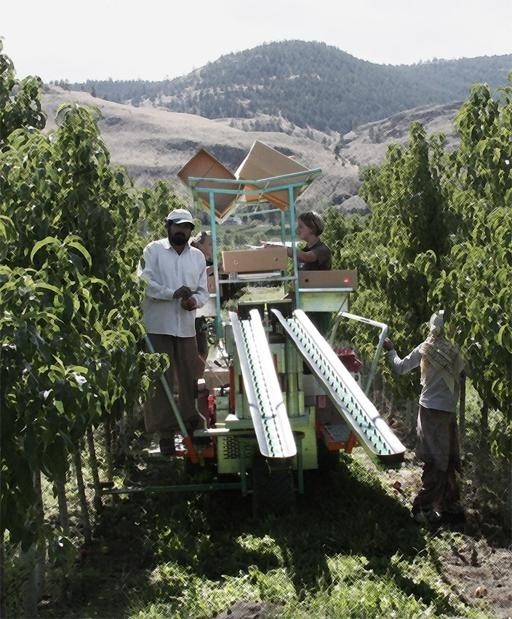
[
  {"xmin": 137, "ymin": 207, "xmax": 212, "ymax": 458},
  {"xmin": 263, "ymin": 211, "xmax": 332, "ymax": 375},
  {"xmin": 382, "ymin": 308, "xmax": 466, "ymax": 519},
  {"xmin": 185, "ymin": 230, "xmax": 212, "ymax": 399}
]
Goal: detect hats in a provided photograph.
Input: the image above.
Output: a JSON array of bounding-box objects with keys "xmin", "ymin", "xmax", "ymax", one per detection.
[{"xmin": 167, "ymin": 209, "xmax": 196, "ymax": 227}]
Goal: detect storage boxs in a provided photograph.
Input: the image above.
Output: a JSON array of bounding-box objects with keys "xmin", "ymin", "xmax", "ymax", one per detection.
[
  {"xmin": 295, "ymin": 268, "xmax": 360, "ymax": 290},
  {"xmin": 175, "ymin": 149, "xmax": 242, "ymax": 223},
  {"xmin": 234, "ymin": 140, "xmax": 314, "ymax": 211},
  {"xmin": 220, "ymin": 246, "xmax": 289, "ymax": 273}
]
